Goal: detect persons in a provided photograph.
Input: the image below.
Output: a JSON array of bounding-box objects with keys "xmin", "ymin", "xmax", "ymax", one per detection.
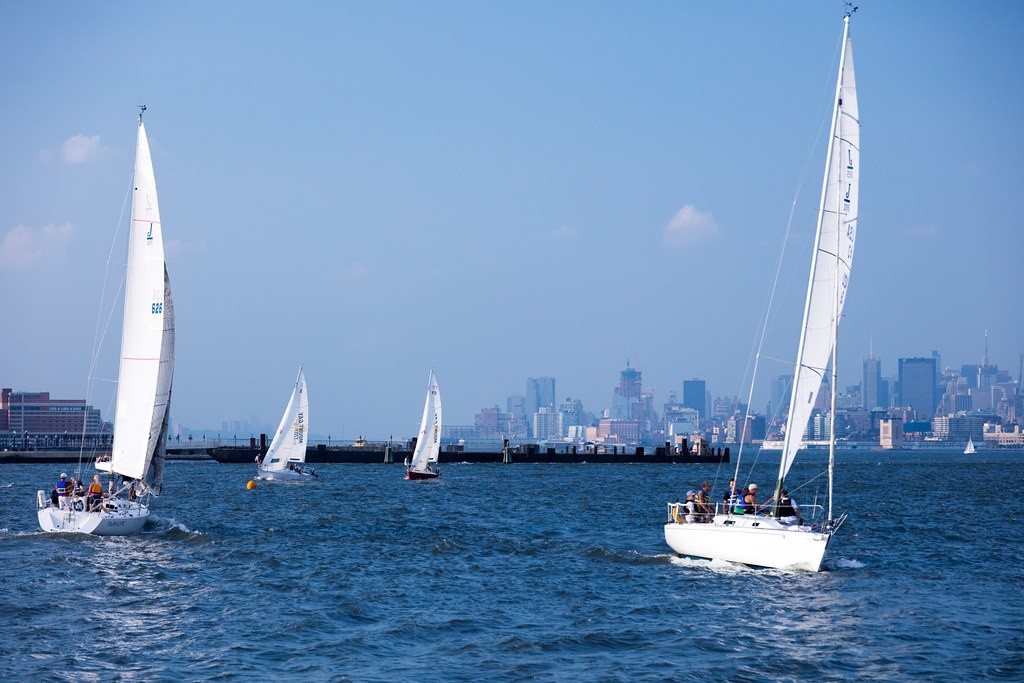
[
  {"xmin": 289, "ymin": 463, "xmax": 302, "ymax": 474},
  {"xmin": 722, "ymin": 478, "xmax": 763, "ymax": 515},
  {"xmin": 403, "ymin": 455, "xmax": 410, "ymax": 470},
  {"xmin": 684, "ymin": 482, "xmax": 717, "ymax": 523},
  {"xmin": 96, "ymin": 455, "xmax": 110, "ymax": 463},
  {"xmin": 255, "ymin": 453, "xmax": 262, "ymax": 466},
  {"xmin": 777, "ymin": 490, "xmax": 799, "ymax": 519},
  {"xmin": 87, "ymin": 474, "xmax": 102, "ymax": 512},
  {"xmin": 50, "ymin": 472, "xmax": 85, "ymax": 511},
  {"xmin": 427, "ymin": 463, "xmax": 432, "ymax": 470}
]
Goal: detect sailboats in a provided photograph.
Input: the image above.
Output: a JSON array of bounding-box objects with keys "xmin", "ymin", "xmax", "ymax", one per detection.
[
  {"xmin": 255, "ymin": 366, "xmax": 319, "ymax": 482},
  {"xmin": 964, "ymin": 436, "xmax": 977, "ymax": 454},
  {"xmin": 36, "ymin": 105, "xmax": 176, "ymax": 535},
  {"xmin": 662, "ymin": 0, "xmax": 862, "ymax": 574},
  {"xmin": 405, "ymin": 369, "xmax": 443, "ymax": 481}
]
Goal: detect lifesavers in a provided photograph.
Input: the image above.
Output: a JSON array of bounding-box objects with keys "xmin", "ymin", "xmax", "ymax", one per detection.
[
  {"xmin": 73, "ymin": 499, "xmax": 84, "ymax": 511},
  {"xmin": 673, "ymin": 508, "xmax": 687, "ymax": 523}
]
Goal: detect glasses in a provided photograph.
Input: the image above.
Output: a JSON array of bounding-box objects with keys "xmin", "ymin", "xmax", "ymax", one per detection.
[{"xmin": 708, "ymin": 486, "xmax": 711, "ymax": 487}]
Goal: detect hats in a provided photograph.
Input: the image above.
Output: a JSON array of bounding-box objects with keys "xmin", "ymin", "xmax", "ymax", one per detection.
[
  {"xmin": 687, "ymin": 491, "xmax": 696, "ymax": 496},
  {"xmin": 93, "ymin": 475, "xmax": 99, "ymax": 479},
  {"xmin": 60, "ymin": 473, "xmax": 68, "ymax": 479},
  {"xmin": 748, "ymin": 483, "xmax": 757, "ymax": 491}
]
